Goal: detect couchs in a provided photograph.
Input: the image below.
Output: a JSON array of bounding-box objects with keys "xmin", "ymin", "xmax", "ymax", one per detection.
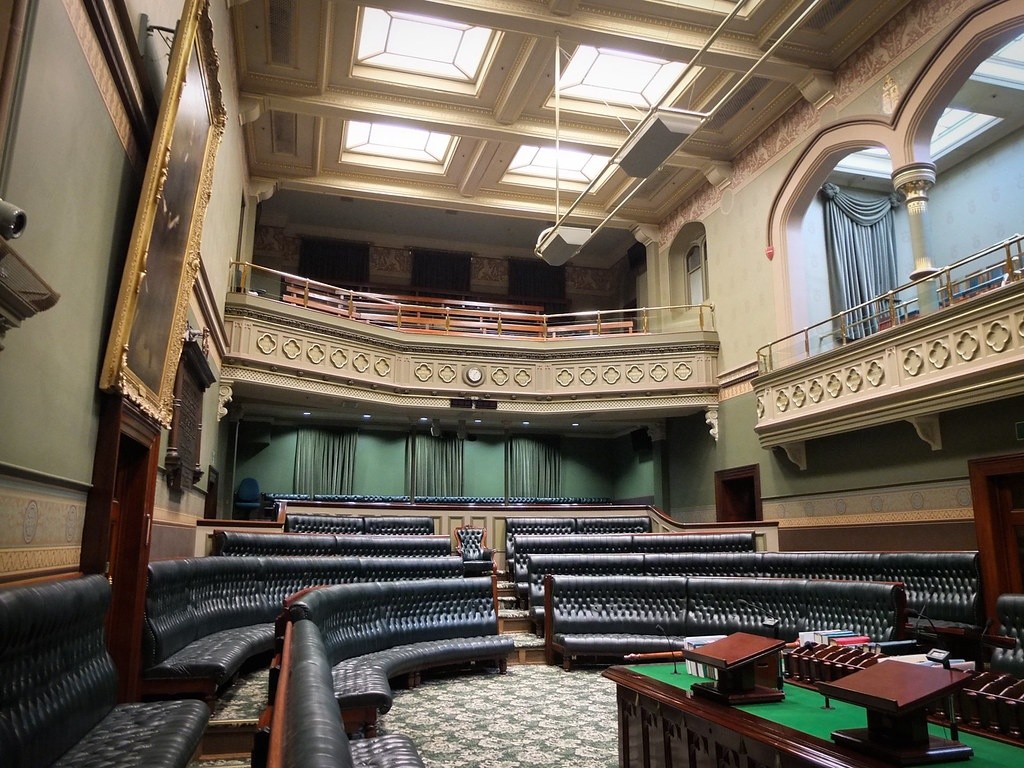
[
  {"xmin": 0, "ymin": 515, "xmax": 514, "ymax": 768},
  {"xmin": 265, "ymin": 492, "xmax": 615, "ymax": 518},
  {"xmin": 503, "ymin": 516, "xmax": 1024, "ymax": 678}
]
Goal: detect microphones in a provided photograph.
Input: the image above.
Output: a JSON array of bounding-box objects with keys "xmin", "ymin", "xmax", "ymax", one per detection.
[
  {"xmin": 656, "ymin": 624, "xmax": 678, "ymax": 673},
  {"xmin": 805, "ymin": 641, "xmax": 826, "ymax": 683},
  {"xmin": 860, "ymin": 606, "xmax": 995, "ymax": 668},
  {"xmin": 736, "ymin": 598, "xmax": 776, "ymax": 622}
]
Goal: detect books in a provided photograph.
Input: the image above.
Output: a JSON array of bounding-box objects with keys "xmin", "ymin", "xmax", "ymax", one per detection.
[
  {"xmin": 681, "ymin": 634, "xmax": 727, "ymax": 681},
  {"xmin": 798, "ymin": 629, "xmax": 976, "ymax": 670}
]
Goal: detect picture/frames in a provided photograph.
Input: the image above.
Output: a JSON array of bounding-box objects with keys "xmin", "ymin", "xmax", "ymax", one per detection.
[{"xmin": 98, "ymin": 0, "xmax": 228, "ymax": 432}]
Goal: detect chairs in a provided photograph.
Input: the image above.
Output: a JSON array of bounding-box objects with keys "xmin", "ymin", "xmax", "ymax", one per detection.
[
  {"xmin": 873, "ymin": 253, "xmax": 1024, "ymax": 331},
  {"xmin": 234, "ymin": 479, "xmax": 267, "ymax": 518},
  {"xmin": 453, "ymin": 527, "xmax": 495, "ymax": 562}
]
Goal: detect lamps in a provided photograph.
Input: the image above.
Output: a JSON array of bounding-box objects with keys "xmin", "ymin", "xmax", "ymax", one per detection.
[
  {"xmin": 618, "ymin": 115, "xmax": 701, "ymax": 178},
  {"xmin": 541, "ymin": 226, "xmax": 592, "ymax": 267}
]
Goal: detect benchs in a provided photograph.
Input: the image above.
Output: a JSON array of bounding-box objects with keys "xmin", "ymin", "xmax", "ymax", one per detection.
[{"xmin": 284, "ymin": 278, "xmax": 633, "ymax": 336}]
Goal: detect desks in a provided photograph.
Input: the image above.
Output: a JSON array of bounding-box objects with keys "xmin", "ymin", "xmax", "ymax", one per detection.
[{"xmin": 601, "ymin": 660, "xmax": 1024, "ymax": 768}]
